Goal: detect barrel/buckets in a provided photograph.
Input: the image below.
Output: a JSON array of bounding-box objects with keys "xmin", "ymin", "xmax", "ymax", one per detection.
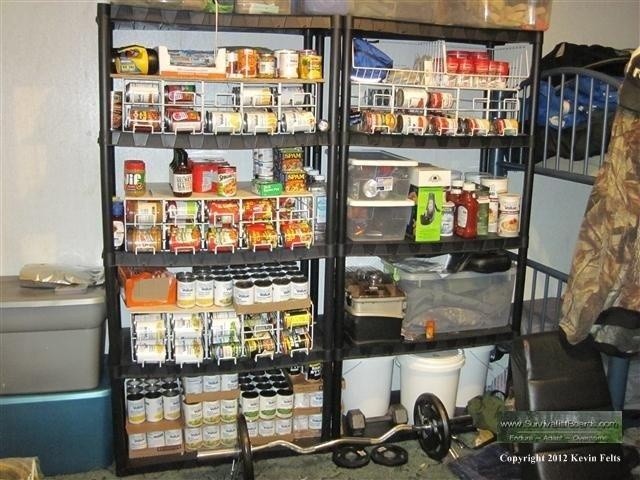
[
  {"xmin": 395, "ymin": 348, "xmax": 465, "ymax": 425},
  {"xmin": 455, "ymin": 345, "xmax": 498, "ymax": 407},
  {"xmin": 340, "ymin": 355, "xmax": 396, "ymax": 422},
  {"xmin": 484, "ymin": 353, "xmax": 511, "ymax": 398}
]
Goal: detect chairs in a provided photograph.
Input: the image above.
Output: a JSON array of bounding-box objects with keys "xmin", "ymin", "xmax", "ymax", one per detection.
[{"xmin": 510, "ymin": 330, "xmax": 630, "ymax": 480}]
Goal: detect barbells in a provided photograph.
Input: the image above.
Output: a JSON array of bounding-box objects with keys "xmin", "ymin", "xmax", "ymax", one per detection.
[{"xmin": 197, "ymin": 393, "xmax": 475, "ymax": 479}]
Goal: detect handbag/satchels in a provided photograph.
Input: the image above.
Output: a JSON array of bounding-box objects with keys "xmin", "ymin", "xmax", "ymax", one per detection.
[{"xmin": 352, "ymin": 36, "xmax": 393, "ymax": 78}]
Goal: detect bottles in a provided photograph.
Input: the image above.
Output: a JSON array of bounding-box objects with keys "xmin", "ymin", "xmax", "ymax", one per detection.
[
  {"xmin": 441, "ymin": 181, "xmax": 479, "ymax": 239},
  {"xmin": 168, "ymin": 148, "xmax": 193, "ymax": 197},
  {"xmin": 304, "ymin": 167, "xmax": 327, "ymax": 243}
]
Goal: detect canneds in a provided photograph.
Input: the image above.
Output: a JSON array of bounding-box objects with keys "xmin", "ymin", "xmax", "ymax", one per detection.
[
  {"xmin": 429, "ymin": 116, "xmax": 465, "ymax": 133},
  {"xmin": 433, "ymin": 49, "xmax": 510, "ymax": 86},
  {"xmin": 440, "ymin": 205, "xmax": 455, "ymax": 237},
  {"xmin": 133, "ymin": 311, "xmax": 313, "ymax": 363},
  {"xmin": 189, "ymin": 155, "xmax": 238, "ymax": 198},
  {"xmin": 464, "ymin": 172, "xmax": 523, "ymax": 236},
  {"xmin": 109, "ymin": 83, "xmax": 318, "ymax": 133},
  {"xmin": 126, "ymin": 199, "xmax": 312, "ymax": 254},
  {"xmin": 362, "ymin": 111, "xmax": 396, "ymax": 133},
  {"xmin": 225, "ymin": 48, "xmax": 322, "ymax": 80},
  {"xmin": 397, "ymin": 115, "xmax": 429, "ymax": 134},
  {"xmin": 397, "ymin": 88, "xmax": 430, "ymax": 108},
  {"xmin": 126, "ymin": 364, "xmax": 324, "ymax": 453},
  {"xmin": 466, "ymin": 119, "xmax": 493, "ymax": 133},
  {"xmin": 124, "ymin": 159, "xmax": 144, "ymax": 196},
  {"xmin": 176, "ymin": 262, "xmax": 309, "ymax": 308},
  {"xmin": 428, "ymin": 92, "xmax": 454, "ymax": 109},
  {"xmin": 252, "ymin": 147, "xmax": 273, "ymax": 179},
  {"xmin": 495, "ymin": 118, "xmax": 519, "ymax": 135}
]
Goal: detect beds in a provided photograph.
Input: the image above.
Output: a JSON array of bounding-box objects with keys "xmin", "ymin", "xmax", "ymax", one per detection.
[{"xmin": 486, "ymin": 63, "xmax": 640, "ymax": 420}]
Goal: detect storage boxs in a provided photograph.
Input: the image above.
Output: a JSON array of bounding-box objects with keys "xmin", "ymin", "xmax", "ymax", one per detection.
[{"xmin": 1, "ymin": 275, "xmax": 115, "ymax": 476}]
